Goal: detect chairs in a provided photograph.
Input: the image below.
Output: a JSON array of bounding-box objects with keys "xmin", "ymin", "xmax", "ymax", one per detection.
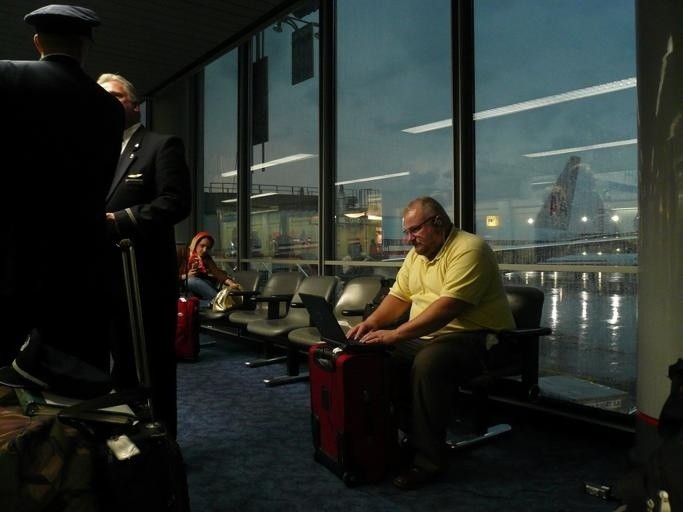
[
  {"xmin": 198, "ymin": 264, "xmax": 399, "ymax": 387},
  {"xmin": 443, "ymin": 284, "xmax": 550, "ymax": 453}
]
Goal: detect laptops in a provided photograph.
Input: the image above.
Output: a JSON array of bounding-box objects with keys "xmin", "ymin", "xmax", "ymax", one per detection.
[{"xmin": 298, "ymin": 292, "xmax": 397, "ymax": 352}]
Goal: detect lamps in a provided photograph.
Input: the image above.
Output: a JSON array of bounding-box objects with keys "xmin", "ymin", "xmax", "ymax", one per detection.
[{"xmin": 273, "ymin": 13, "xmax": 320, "ymax": 41}]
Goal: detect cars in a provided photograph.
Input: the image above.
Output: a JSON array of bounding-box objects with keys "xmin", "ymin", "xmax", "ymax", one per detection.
[{"xmin": 230, "ymin": 245, "xmax": 261, "ymax": 257}]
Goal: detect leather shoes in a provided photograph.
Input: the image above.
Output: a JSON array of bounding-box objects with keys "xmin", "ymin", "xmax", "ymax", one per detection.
[{"xmin": 391, "ymin": 457, "xmax": 451, "ymax": 490}]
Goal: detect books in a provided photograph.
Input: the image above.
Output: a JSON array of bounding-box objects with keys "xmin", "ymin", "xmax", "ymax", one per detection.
[{"xmin": 13, "ymin": 387, "xmax": 141, "ymax": 425}]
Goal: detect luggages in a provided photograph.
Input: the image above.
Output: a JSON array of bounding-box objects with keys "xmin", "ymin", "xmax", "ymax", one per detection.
[
  {"xmin": 176, "ymin": 295, "xmax": 202, "ymax": 363},
  {"xmin": 305, "ymin": 342, "xmax": 401, "ymax": 489}
]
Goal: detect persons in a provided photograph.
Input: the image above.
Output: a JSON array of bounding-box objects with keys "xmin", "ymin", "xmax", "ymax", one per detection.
[
  {"xmin": 95, "ymin": 71, "xmax": 192, "ymax": 439},
  {"xmin": 0, "ymin": 4, "xmax": 126, "ymax": 367},
  {"xmin": 345, "ymin": 196, "xmax": 521, "ymax": 491},
  {"xmin": 177, "ymin": 231, "xmax": 241, "ymax": 300}
]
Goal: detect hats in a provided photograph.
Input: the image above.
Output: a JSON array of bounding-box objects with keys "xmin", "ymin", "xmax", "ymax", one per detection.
[
  {"xmin": 21, "ymin": 3, "xmax": 104, "ymax": 37},
  {"xmin": 0, "ymin": 324, "xmax": 79, "ymax": 392}
]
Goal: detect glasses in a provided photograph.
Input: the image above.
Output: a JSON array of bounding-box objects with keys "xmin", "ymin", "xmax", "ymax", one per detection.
[{"xmin": 403, "ymin": 213, "xmax": 439, "ymax": 235}]
